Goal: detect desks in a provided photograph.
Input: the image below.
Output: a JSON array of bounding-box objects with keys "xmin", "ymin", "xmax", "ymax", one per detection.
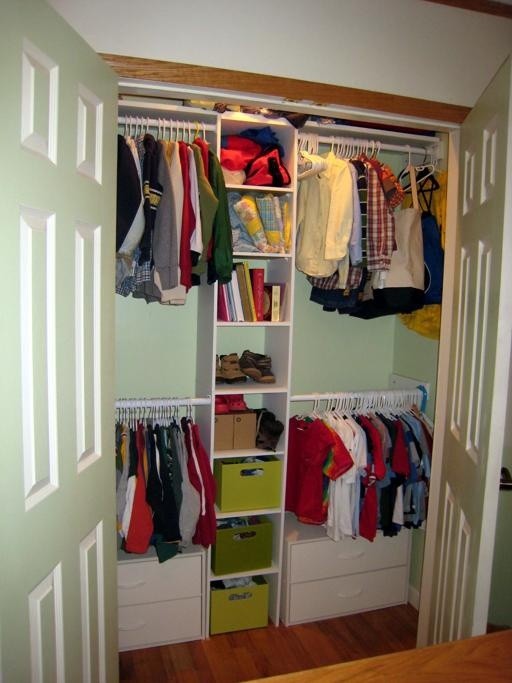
[{"xmin": 263, "ymin": 626, "xmax": 512, "ymax": 683}]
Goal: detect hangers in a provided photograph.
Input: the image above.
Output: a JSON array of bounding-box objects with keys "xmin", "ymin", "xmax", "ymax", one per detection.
[
  {"xmin": 295, "ymin": 388, "xmax": 427, "ymax": 429},
  {"xmin": 297, "ymin": 130, "xmax": 444, "ymax": 193},
  {"xmin": 115, "ymin": 396, "xmax": 202, "ymax": 439},
  {"xmin": 118, "ymin": 113, "xmax": 210, "ymax": 153}
]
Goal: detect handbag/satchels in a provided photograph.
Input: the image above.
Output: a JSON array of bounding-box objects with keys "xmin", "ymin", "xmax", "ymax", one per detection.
[
  {"xmin": 373, "ymin": 163, "xmax": 444, "ymax": 310},
  {"xmin": 249, "ymin": 407, "xmax": 284, "ymax": 450}
]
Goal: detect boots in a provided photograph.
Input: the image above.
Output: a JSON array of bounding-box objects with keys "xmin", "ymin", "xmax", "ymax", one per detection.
[{"xmin": 217, "ymin": 350, "xmax": 247, "ymax": 384}]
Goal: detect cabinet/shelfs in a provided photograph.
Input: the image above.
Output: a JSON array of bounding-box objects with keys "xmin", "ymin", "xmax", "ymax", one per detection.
[
  {"xmin": 282, "ymin": 512, "xmax": 413, "ymax": 626},
  {"xmin": 197, "ymin": 109, "xmax": 296, "ymax": 636},
  {"xmin": 118, "ymin": 542, "xmax": 206, "ymax": 654}
]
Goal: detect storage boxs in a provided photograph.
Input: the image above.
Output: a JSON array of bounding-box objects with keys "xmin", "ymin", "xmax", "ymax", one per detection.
[{"xmin": 210, "ymin": 409, "xmax": 284, "ymax": 635}]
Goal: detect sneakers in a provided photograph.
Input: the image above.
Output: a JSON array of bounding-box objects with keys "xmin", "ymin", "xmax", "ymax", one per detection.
[
  {"xmin": 214, "ymin": 394, "xmax": 247, "ymax": 415},
  {"xmin": 239, "ymin": 349, "xmax": 275, "ymax": 384}
]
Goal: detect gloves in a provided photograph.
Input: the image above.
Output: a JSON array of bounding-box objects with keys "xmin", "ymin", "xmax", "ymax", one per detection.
[{"xmin": 244, "ymin": 145, "xmax": 292, "ymax": 186}]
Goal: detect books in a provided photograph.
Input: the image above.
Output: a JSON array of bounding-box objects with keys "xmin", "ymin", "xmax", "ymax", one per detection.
[{"xmin": 218, "ymin": 261, "xmax": 285, "ymax": 321}]
